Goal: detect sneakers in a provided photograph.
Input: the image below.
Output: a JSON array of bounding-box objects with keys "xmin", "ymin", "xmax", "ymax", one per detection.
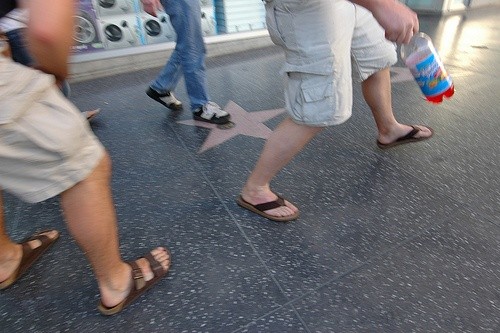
[
  {"xmin": 145, "ymin": 86, "xmax": 183, "ymax": 111},
  {"xmin": 192, "ymin": 100, "xmax": 231, "ymax": 124}
]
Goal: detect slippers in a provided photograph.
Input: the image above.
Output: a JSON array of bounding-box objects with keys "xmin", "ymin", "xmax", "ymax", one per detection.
[
  {"xmin": 377, "ymin": 124, "xmax": 434, "ymax": 149},
  {"xmin": 237, "ymin": 193, "xmax": 300, "ymax": 221},
  {"xmin": 97, "ymin": 246, "xmax": 171, "ymax": 315},
  {"xmin": 0, "ymin": 228, "xmax": 60, "ymax": 290}
]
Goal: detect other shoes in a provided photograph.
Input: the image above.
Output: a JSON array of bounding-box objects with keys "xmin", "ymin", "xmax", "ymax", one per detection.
[{"xmin": 81, "ymin": 108, "xmax": 101, "ymax": 121}]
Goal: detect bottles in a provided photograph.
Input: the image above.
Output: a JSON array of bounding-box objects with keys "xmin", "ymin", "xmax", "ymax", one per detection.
[{"xmin": 400, "ymin": 32, "xmax": 454, "ymax": 103}]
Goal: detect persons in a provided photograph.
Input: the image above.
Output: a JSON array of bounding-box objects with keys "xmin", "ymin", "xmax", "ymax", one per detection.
[
  {"xmin": 235, "ymin": 0, "xmax": 434, "ymax": 222},
  {"xmin": 0, "ymin": 0, "xmax": 171, "ymax": 315},
  {"xmin": 141, "ymin": 0, "xmax": 231, "ymax": 125}
]
